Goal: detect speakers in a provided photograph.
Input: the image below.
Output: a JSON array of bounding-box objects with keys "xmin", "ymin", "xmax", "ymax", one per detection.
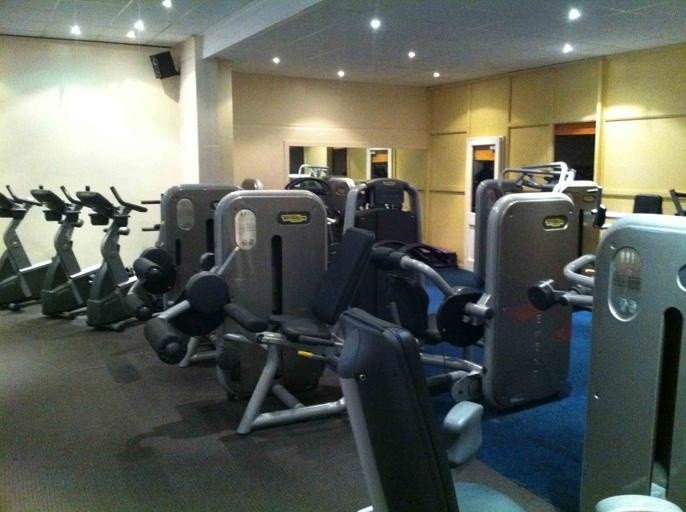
[{"xmin": 150, "ymin": 51, "xmax": 179, "ymax": 81}]
